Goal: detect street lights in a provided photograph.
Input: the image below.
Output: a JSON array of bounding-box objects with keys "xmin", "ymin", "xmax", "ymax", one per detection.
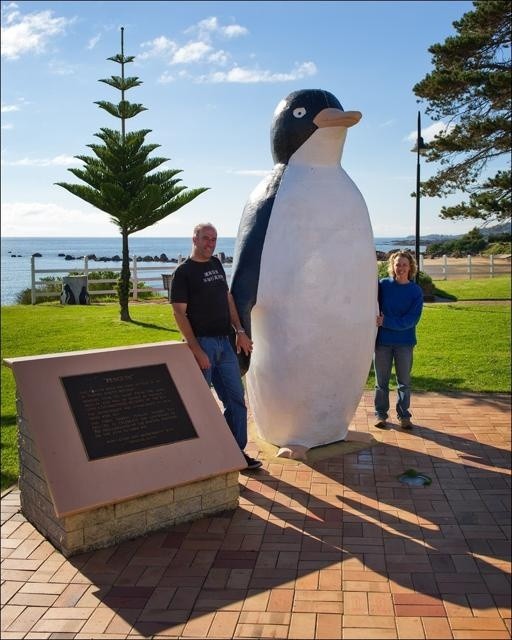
[{"xmin": 409, "ymin": 110, "xmax": 433, "ymax": 288}]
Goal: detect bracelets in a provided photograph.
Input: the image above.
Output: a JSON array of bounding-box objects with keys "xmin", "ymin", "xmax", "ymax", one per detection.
[{"xmin": 234, "ymin": 328, "xmax": 245, "ymax": 334}]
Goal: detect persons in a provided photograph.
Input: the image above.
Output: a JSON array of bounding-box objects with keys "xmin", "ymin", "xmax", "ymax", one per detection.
[
  {"xmin": 374, "ymin": 252, "xmax": 421, "ymax": 429},
  {"xmin": 169, "ymin": 223, "xmax": 262, "ymax": 469}
]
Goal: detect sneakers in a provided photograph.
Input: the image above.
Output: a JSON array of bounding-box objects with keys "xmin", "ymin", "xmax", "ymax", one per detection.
[
  {"xmin": 375, "ymin": 417, "xmax": 386, "ymax": 426},
  {"xmin": 399, "ymin": 417, "xmax": 412, "ymax": 428},
  {"xmin": 241, "ymin": 450, "xmax": 262, "ymax": 469}
]
